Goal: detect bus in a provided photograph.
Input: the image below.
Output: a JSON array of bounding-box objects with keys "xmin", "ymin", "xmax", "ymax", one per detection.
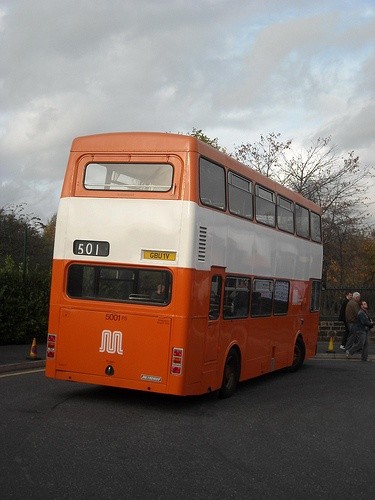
[{"xmin": 44, "ymin": 132, "xmax": 327, "ymax": 400}]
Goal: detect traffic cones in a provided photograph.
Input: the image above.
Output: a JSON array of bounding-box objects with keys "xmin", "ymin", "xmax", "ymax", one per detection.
[
  {"xmin": 26, "ymin": 337, "xmax": 41, "ymax": 361},
  {"xmin": 327, "ymin": 336, "xmax": 336, "ymax": 353}
]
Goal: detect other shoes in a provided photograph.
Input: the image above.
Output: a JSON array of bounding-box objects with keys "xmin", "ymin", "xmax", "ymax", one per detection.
[
  {"xmin": 361, "ymin": 359, "xmax": 372, "ymax": 362},
  {"xmin": 344, "ymin": 350, "xmax": 351, "ymax": 359},
  {"xmin": 340, "ymin": 345, "xmax": 345, "ymax": 351}
]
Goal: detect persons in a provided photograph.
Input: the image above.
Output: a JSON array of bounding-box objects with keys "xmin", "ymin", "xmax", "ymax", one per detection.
[
  {"xmin": 339, "ymin": 291, "xmax": 361, "ymax": 351},
  {"xmin": 151, "ymin": 280, "xmax": 166, "ymax": 301},
  {"xmin": 231, "ymin": 280, "xmax": 254, "ymax": 316},
  {"xmin": 345, "ymin": 300, "xmax": 374, "ymax": 362}
]
